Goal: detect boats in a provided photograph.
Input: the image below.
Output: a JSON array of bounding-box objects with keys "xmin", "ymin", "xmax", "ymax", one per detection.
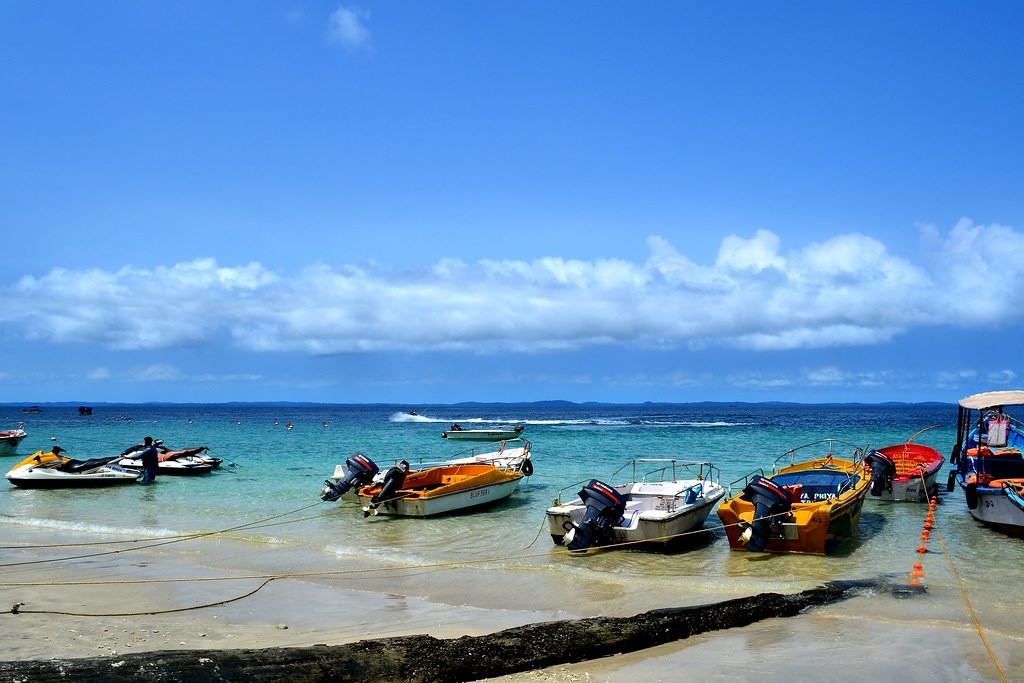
[
  {"xmin": 717, "ymin": 437, "xmax": 877, "ymax": 555},
  {"xmin": 323, "ymin": 436, "xmax": 537, "ymax": 519},
  {"xmin": 546, "ymin": 454, "xmax": 725, "ymax": 550},
  {"xmin": 441, "ymin": 423, "xmax": 525, "ymax": 440},
  {"xmin": 948, "ymin": 388, "xmax": 1023, "ymax": 531},
  {"xmin": 0, "ymin": 421, "xmax": 27, "ymax": 458},
  {"xmin": 865, "ymin": 441, "xmax": 944, "ymax": 502}
]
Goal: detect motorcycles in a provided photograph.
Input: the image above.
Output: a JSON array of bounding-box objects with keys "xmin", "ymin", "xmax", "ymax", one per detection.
[
  {"xmin": 117, "ymin": 439, "xmax": 222, "ymax": 476},
  {"xmin": 4, "ymin": 445, "xmax": 143, "ymax": 488}
]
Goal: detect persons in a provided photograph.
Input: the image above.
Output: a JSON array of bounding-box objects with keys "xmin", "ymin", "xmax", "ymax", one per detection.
[{"xmin": 120, "ymin": 435, "xmax": 160, "ymax": 485}]
